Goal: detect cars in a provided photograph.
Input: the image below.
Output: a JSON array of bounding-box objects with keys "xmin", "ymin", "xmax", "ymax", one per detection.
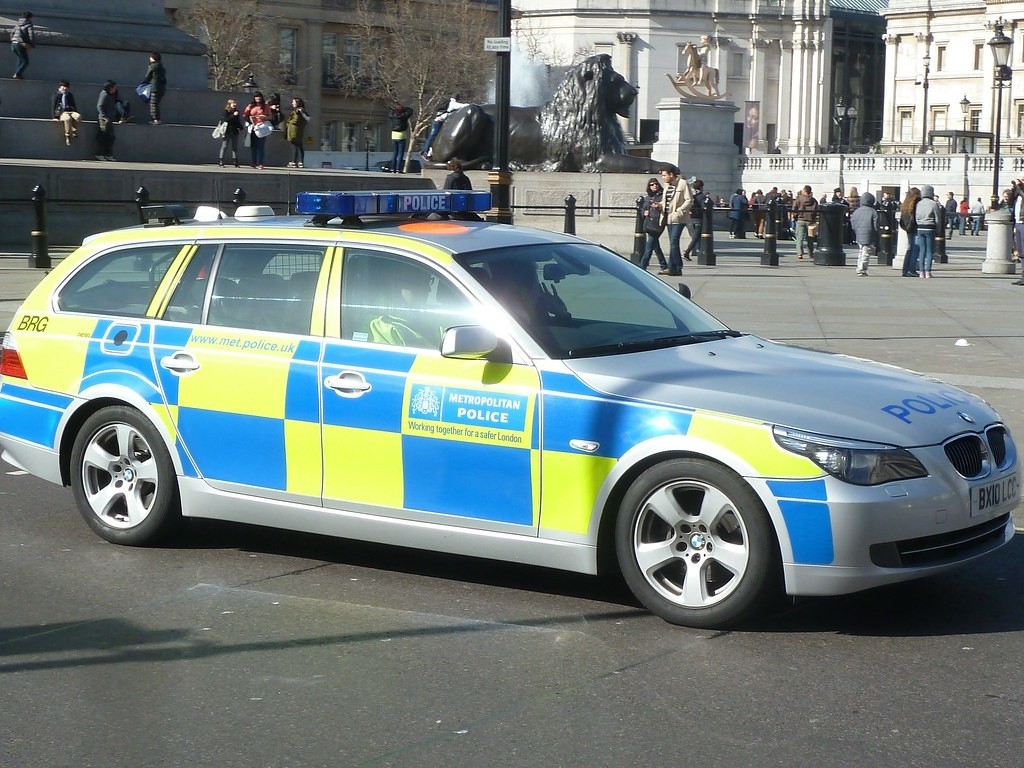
[{"xmin": 0, "ymin": 188, "xmax": 1024, "ymax": 631}]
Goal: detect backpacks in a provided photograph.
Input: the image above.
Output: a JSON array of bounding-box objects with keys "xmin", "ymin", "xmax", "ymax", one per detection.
[{"xmin": 434, "ymin": 99, "xmax": 456, "ymax": 114}]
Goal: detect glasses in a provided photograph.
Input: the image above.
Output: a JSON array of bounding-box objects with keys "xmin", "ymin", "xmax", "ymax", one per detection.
[
  {"xmin": 649, "ymin": 183, "xmax": 658, "ymax": 186},
  {"xmin": 398, "ymin": 277, "xmax": 434, "ymax": 290}
]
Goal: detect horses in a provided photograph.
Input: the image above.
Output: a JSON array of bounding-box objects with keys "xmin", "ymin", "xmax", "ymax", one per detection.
[{"xmin": 677, "ymin": 40, "xmax": 720, "ymax": 97}]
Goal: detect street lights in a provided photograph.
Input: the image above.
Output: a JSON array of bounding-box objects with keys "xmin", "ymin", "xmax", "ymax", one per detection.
[
  {"xmin": 831, "ymin": 96, "xmax": 860, "ymax": 196},
  {"xmin": 960, "ymin": 95, "xmax": 972, "ymax": 202},
  {"xmin": 986, "ymin": 15, "xmax": 1015, "ymax": 208}
]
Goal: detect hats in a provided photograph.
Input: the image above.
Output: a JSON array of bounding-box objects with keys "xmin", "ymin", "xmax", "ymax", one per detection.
[
  {"xmin": 861, "ymin": 192, "xmax": 875, "ymax": 206},
  {"xmin": 921, "ymin": 185, "xmax": 934, "ymax": 199}
]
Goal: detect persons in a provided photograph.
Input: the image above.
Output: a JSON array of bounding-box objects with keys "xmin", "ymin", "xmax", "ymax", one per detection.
[
  {"xmin": 639, "ymin": 178, "xmax": 667, "ymax": 270},
  {"xmin": 959, "ymin": 146, "xmax": 968, "ymax": 153},
  {"xmin": 926, "ymin": 147, "xmax": 934, "ymax": 154},
  {"xmin": 772, "ymin": 148, "xmax": 781, "ymax": 154},
  {"xmin": 368, "ymin": 264, "xmax": 450, "ymax": 348},
  {"xmin": 114, "ymin": 100, "xmax": 134, "ymax": 124},
  {"xmin": 692, "ymin": 34, "xmax": 713, "ymax": 86},
  {"xmin": 506, "ymin": 262, "xmax": 570, "ymax": 323},
  {"xmin": 704, "ymin": 178, "xmax": 1024, "ymax": 286},
  {"xmin": 145, "ymin": 52, "xmax": 167, "ymax": 125},
  {"xmin": 652, "ymin": 166, "xmax": 693, "ymax": 274},
  {"xmin": 52, "ymin": 79, "xmax": 81, "ymax": 146},
  {"xmin": 684, "ymin": 180, "xmax": 706, "ymax": 261},
  {"xmin": 747, "ymin": 104, "xmax": 759, "ymax": 148},
  {"xmin": 266, "ymin": 92, "xmax": 285, "ymax": 130},
  {"xmin": 243, "ymin": 91, "xmax": 272, "ymax": 170},
  {"xmin": 444, "ymin": 161, "xmax": 472, "ymax": 190},
  {"xmin": 418, "ymin": 93, "xmax": 470, "ymax": 162},
  {"xmin": 219, "ymin": 99, "xmax": 245, "ymax": 168},
  {"xmin": 389, "ymin": 106, "xmax": 413, "ymax": 175},
  {"xmin": 10, "ymin": 11, "xmax": 35, "ymax": 80},
  {"xmin": 284, "ymin": 97, "xmax": 306, "ymax": 168},
  {"xmin": 96, "ymin": 80, "xmax": 117, "ymax": 162}
]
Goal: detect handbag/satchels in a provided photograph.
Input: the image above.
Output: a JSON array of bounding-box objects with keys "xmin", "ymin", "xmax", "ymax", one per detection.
[
  {"xmin": 242, "ymin": 124, "xmax": 253, "ymax": 147},
  {"xmin": 657, "ymin": 268, "xmax": 671, "ymax": 274},
  {"xmin": 254, "ymin": 121, "xmax": 274, "ymax": 138},
  {"xmin": 135, "ymin": 83, "xmax": 152, "ymax": 103},
  {"xmin": 212, "ymin": 121, "xmax": 228, "ymax": 139},
  {"xmin": 644, "ymin": 216, "xmax": 665, "ymax": 238}
]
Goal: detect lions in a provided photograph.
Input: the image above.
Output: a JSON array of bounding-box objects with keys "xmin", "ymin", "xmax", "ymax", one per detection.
[{"xmin": 420, "ymin": 55, "xmax": 681, "ymax": 175}]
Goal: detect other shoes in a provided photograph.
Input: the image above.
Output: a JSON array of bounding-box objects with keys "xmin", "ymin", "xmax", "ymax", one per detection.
[
  {"xmin": 121, "ymin": 116, "xmax": 135, "ymax": 122},
  {"xmin": 65, "ymin": 130, "xmax": 77, "ymax": 146},
  {"xmin": 219, "ymin": 163, "xmax": 224, "ymax": 168},
  {"xmin": 857, "ymin": 271, "xmax": 868, "ymax": 276},
  {"xmin": 755, "ymin": 234, "xmax": 765, "ymax": 239},
  {"xmin": 422, "ymin": 152, "xmax": 432, "ymax": 163},
  {"xmin": 729, "ymin": 235, "xmax": 736, "ymax": 239},
  {"xmin": 392, "ymin": 170, "xmax": 404, "ymax": 174},
  {"xmin": 1011, "ymin": 280, "xmax": 1024, "ymax": 285},
  {"xmin": 95, "ymin": 155, "xmax": 116, "ymax": 161},
  {"xmin": 13, "ymin": 74, "xmax": 25, "ymax": 79},
  {"xmin": 149, "ymin": 120, "xmax": 161, "ymax": 124},
  {"xmin": 920, "ymin": 272, "xmax": 932, "ymax": 278},
  {"xmin": 902, "ymin": 270, "xmax": 920, "ymax": 277},
  {"xmin": 682, "ymin": 254, "xmax": 691, "ymax": 261},
  {"xmin": 234, "ymin": 163, "xmax": 240, "ymax": 168},
  {"xmin": 251, "ymin": 164, "xmax": 263, "ymax": 169}
]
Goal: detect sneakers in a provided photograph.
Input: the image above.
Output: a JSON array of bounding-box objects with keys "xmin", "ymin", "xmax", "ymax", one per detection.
[{"xmin": 286, "ymin": 162, "xmax": 303, "ymax": 168}]
